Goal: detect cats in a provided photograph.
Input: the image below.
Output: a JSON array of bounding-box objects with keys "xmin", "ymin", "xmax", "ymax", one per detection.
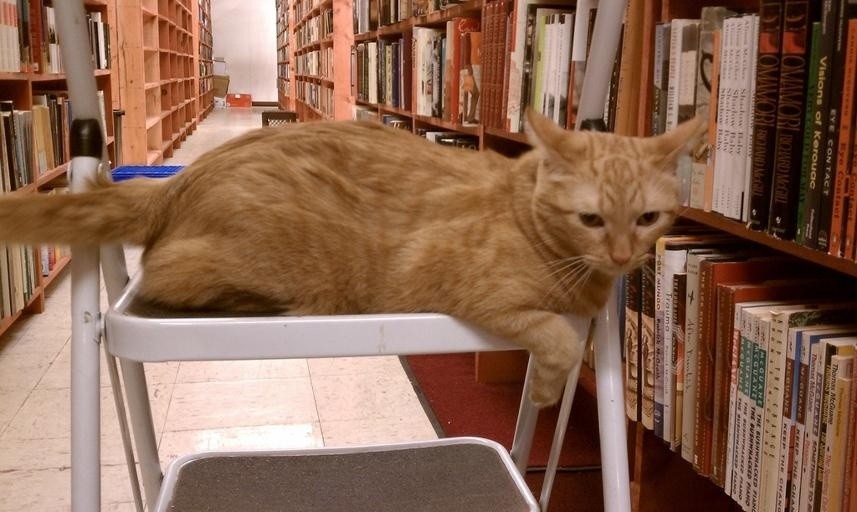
[{"xmin": 0, "ymin": 106, "xmax": 701, "ymax": 406}]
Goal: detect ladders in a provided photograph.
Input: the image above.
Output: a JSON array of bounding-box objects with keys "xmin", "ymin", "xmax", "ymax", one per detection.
[{"xmin": 51, "ymin": 1, "xmax": 631, "ymax": 512}]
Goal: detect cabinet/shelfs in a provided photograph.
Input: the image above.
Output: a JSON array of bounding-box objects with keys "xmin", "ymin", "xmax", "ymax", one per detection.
[
  {"xmin": 275, "ymin": 1, "xmax": 857, "ymax": 512},
  {"xmin": 1, "ymin": 1, "xmax": 213, "ymax": 347}
]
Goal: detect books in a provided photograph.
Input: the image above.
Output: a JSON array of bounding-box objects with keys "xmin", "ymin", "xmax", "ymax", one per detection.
[
  {"xmin": 0, "ymin": 92, "xmax": 70, "ymax": 192},
  {"xmin": 0, "ymin": 0, "xmax": 111, "ymax": 75},
  {"xmin": 276, "ymin": 1, "xmax": 333, "ymax": 119},
  {"xmin": 1, "ymin": 190, "xmax": 71, "ymax": 320},
  {"xmin": 198, "ymin": 0, "xmax": 214, "ymax": 97}
]
